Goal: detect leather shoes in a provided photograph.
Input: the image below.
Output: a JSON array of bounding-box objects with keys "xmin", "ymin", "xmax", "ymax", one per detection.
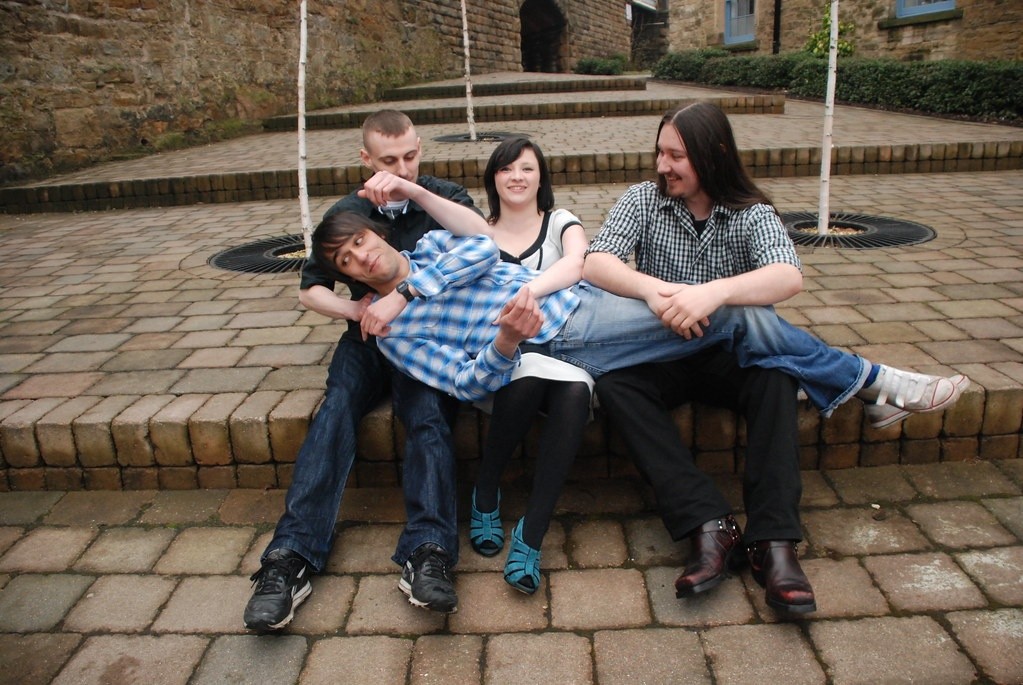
[
  {"xmin": 675, "ymin": 515, "xmax": 747, "ymax": 599},
  {"xmin": 750, "ymin": 540, "xmax": 817, "ymax": 613}
]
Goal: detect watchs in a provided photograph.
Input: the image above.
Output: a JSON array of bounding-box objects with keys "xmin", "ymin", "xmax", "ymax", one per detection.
[{"xmin": 397, "ymin": 282, "xmax": 417, "ymax": 305}]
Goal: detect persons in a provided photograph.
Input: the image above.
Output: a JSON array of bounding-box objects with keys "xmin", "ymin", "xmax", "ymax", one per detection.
[
  {"xmin": 238, "ymin": 110, "xmax": 495, "ymax": 637},
  {"xmin": 312, "ymin": 208, "xmax": 973, "ymax": 433},
  {"xmin": 576, "ymin": 96, "xmax": 819, "ymax": 614},
  {"xmin": 470, "ymin": 138, "xmax": 592, "ymax": 602}
]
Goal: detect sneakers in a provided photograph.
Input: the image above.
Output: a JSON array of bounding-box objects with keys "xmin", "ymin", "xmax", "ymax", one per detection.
[
  {"xmin": 854, "ymin": 361, "xmax": 959, "ymax": 414},
  {"xmin": 865, "ymin": 375, "xmax": 969, "ymax": 432},
  {"xmin": 243, "ymin": 547, "xmax": 313, "ymax": 630},
  {"xmin": 397, "ymin": 542, "xmax": 458, "ymax": 613}
]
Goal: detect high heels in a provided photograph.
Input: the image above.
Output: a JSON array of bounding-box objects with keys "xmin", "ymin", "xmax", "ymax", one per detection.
[
  {"xmin": 471, "ymin": 481, "xmax": 504, "ymax": 557},
  {"xmin": 502, "ymin": 516, "xmax": 543, "ymax": 594}
]
